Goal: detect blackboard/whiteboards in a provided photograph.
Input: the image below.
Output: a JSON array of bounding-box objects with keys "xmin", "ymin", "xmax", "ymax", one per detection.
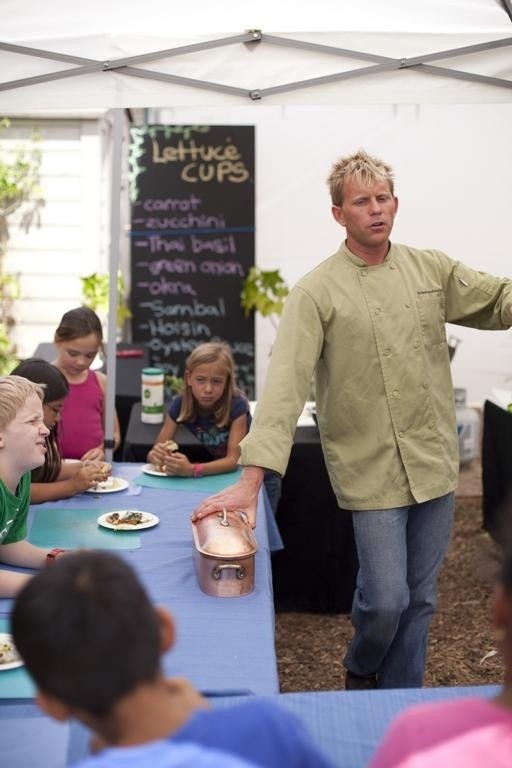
[{"xmin": 128, "ymin": 124, "xmax": 256, "ymax": 402}]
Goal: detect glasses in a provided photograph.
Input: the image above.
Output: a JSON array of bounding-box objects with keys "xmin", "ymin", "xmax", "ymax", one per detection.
[{"xmin": 43, "ymin": 402, "xmax": 63, "ymax": 416}]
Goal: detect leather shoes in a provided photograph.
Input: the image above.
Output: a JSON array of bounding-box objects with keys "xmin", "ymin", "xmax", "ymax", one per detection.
[{"xmin": 344, "ymin": 668, "xmax": 377, "ymax": 691}]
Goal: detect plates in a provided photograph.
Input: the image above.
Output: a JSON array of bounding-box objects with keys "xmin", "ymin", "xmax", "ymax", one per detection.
[
  {"xmin": 0, "ymin": 632, "xmax": 26, "ymax": 671},
  {"xmin": 141, "ymin": 462, "xmax": 172, "ymax": 479},
  {"xmin": 96, "ymin": 509, "xmax": 161, "ymax": 534},
  {"xmin": 85, "ymin": 475, "xmax": 130, "ymax": 494}
]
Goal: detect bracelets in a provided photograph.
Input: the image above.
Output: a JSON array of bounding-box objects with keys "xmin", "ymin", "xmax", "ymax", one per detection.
[
  {"xmin": 193, "ymin": 462, "xmax": 203, "ymax": 478},
  {"xmin": 46, "ymin": 549, "xmax": 64, "ymax": 565}
]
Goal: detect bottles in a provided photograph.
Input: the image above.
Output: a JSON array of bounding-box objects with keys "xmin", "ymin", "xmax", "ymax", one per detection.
[{"xmin": 141, "ymin": 367, "xmax": 165, "ymax": 425}]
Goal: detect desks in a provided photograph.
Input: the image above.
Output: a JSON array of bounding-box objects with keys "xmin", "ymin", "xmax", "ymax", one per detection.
[
  {"xmin": 1, "ymin": 681, "xmax": 512, "ymax": 767},
  {"xmin": 0, "ymin": 458, "xmax": 283, "ymax": 698},
  {"xmin": 0, "ymin": 338, "xmax": 511, "ymax": 613}
]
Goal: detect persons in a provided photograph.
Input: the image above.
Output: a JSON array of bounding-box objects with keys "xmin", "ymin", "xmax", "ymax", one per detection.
[
  {"xmin": 145, "ymin": 342, "xmax": 282, "ymax": 519},
  {"xmin": 48, "ymin": 305, "xmax": 122, "ymax": 463},
  {"xmin": 10, "ymin": 546, "xmax": 330, "ymax": 767},
  {"xmin": 0, "ymin": 375, "xmax": 65, "ymax": 601},
  {"xmin": 190, "ymin": 149, "xmax": 511, "ymax": 691},
  {"xmin": 360, "ymin": 507, "xmax": 512, "ymax": 767},
  {"xmin": 9, "ymin": 357, "xmax": 104, "ymax": 505}
]
